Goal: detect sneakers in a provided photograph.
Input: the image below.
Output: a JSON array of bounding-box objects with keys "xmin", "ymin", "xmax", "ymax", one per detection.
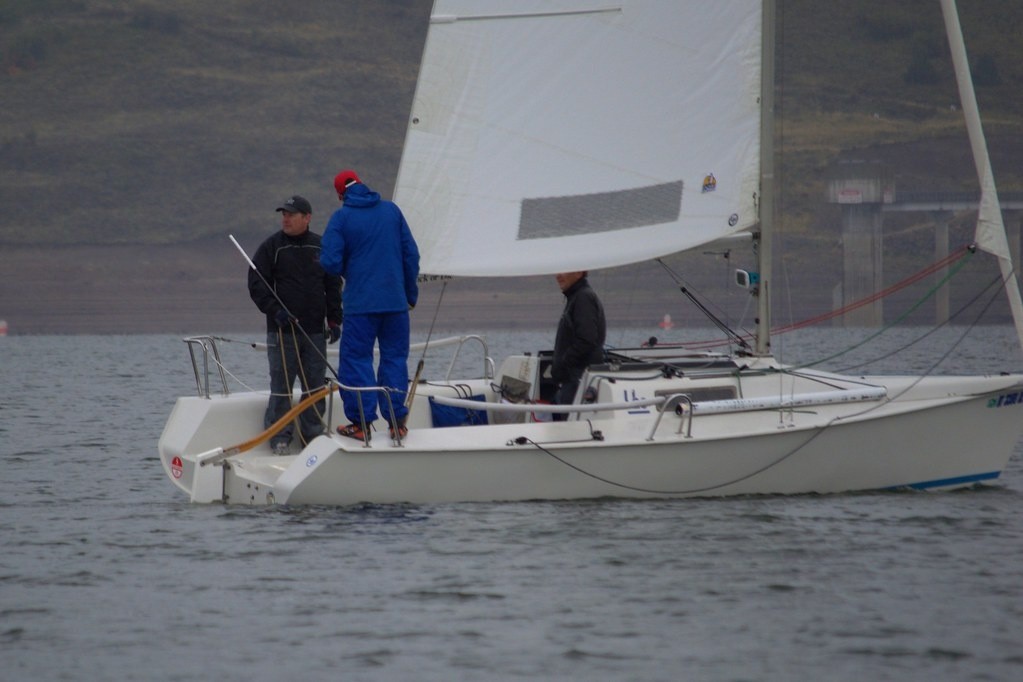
[
  {"xmin": 272, "ymin": 442, "xmax": 290, "ymax": 455},
  {"xmin": 336, "ymin": 424, "xmax": 371, "ymax": 441},
  {"xmin": 389, "ymin": 425, "xmax": 408, "ymax": 440}
]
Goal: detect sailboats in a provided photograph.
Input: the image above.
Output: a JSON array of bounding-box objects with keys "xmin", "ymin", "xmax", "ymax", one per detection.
[{"xmin": 159, "ymin": 0, "xmax": 1023, "ymax": 506}]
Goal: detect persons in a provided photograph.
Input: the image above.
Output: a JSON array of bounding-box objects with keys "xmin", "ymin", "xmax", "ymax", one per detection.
[
  {"xmin": 318, "ymin": 170, "xmax": 421, "ymax": 440},
  {"xmin": 248, "ymin": 194, "xmax": 344, "ymax": 456},
  {"xmin": 548, "ymin": 269, "xmax": 607, "ymax": 422}
]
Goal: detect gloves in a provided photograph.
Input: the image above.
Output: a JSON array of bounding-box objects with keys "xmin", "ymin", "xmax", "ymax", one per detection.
[
  {"xmin": 275, "ymin": 311, "xmax": 299, "ymax": 335},
  {"xmin": 324, "ymin": 323, "xmax": 340, "ymax": 344}
]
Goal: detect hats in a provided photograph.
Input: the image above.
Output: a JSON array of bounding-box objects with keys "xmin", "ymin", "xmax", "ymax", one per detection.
[
  {"xmin": 274, "ymin": 196, "xmax": 311, "ymax": 215},
  {"xmin": 334, "ymin": 171, "xmax": 362, "ymax": 193}
]
